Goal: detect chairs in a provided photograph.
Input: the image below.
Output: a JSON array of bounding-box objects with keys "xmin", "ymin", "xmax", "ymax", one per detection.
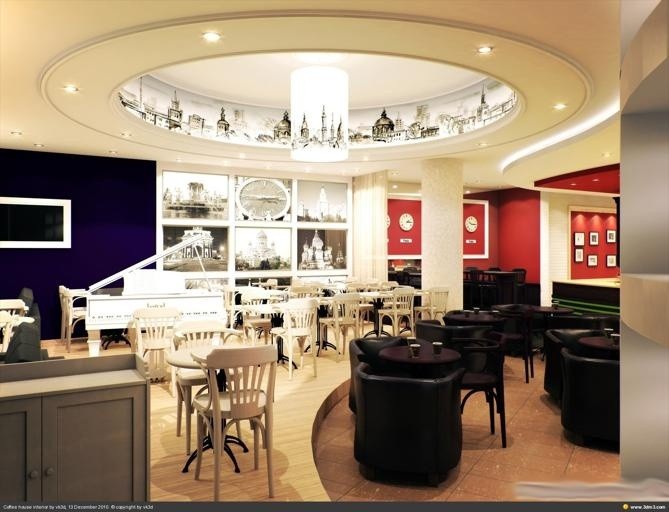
[{"xmin": 0, "ymin": 262, "xmax": 623, "ymax": 502}]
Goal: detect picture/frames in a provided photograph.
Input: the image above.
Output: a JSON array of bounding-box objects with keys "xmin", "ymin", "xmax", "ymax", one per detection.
[
  {"xmin": 1, "ymin": 195, "xmax": 74, "ymax": 249},
  {"xmin": 574, "ymin": 227, "xmax": 617, "ymax": 268}
]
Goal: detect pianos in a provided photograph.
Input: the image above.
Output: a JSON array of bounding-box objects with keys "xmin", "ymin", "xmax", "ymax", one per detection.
[{"xmin": 84, "ymin": 230, "xmax": 228, "ymax": 356}]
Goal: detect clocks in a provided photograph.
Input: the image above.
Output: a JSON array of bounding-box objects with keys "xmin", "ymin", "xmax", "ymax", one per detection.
[
  {"xmin": 464, "ymin": 214, "xmax": 478, "ymax": 233},
  {"xmin": 234, "ymin": 175, "xmax": 295, "ymax": 221},
  {"xmin": 399, "ymin": 211, "xmax": 415, "ymax": 232}
]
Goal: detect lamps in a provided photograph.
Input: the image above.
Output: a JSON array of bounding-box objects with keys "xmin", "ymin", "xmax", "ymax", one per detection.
[{"xmin": 288, "ymin": 64, "xmax": 350, "ymax": 160}]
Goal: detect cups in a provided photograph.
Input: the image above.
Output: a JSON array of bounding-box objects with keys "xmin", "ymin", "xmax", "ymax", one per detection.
[
  {"xmin": 553, "ymin": 303, "xmax": 558, "ymax": 310},
  {"xmin": 409, "ymin": 343, "xmax": 421, "ymax": 359},
  {"xmin": 406, "ymin": 336, "xmax": 416, "ymax": 345},
  {"xmin": 432, "ymin": 342, "xmax": 443, "ymax": 356},
  {"xmin": 609, "ymin": 333, "xmax": 620, "ymax": 346},
  {"xmin": 603, "ymin": 328, "xmax": 614, "ymax": 339},
  {"xmin": 474, "ymin": 307, "xmax": 480, "ymax": 316},
  {"xmin": 464, "ymin": 310, "xmax": 470, "ymax": 319}
]
